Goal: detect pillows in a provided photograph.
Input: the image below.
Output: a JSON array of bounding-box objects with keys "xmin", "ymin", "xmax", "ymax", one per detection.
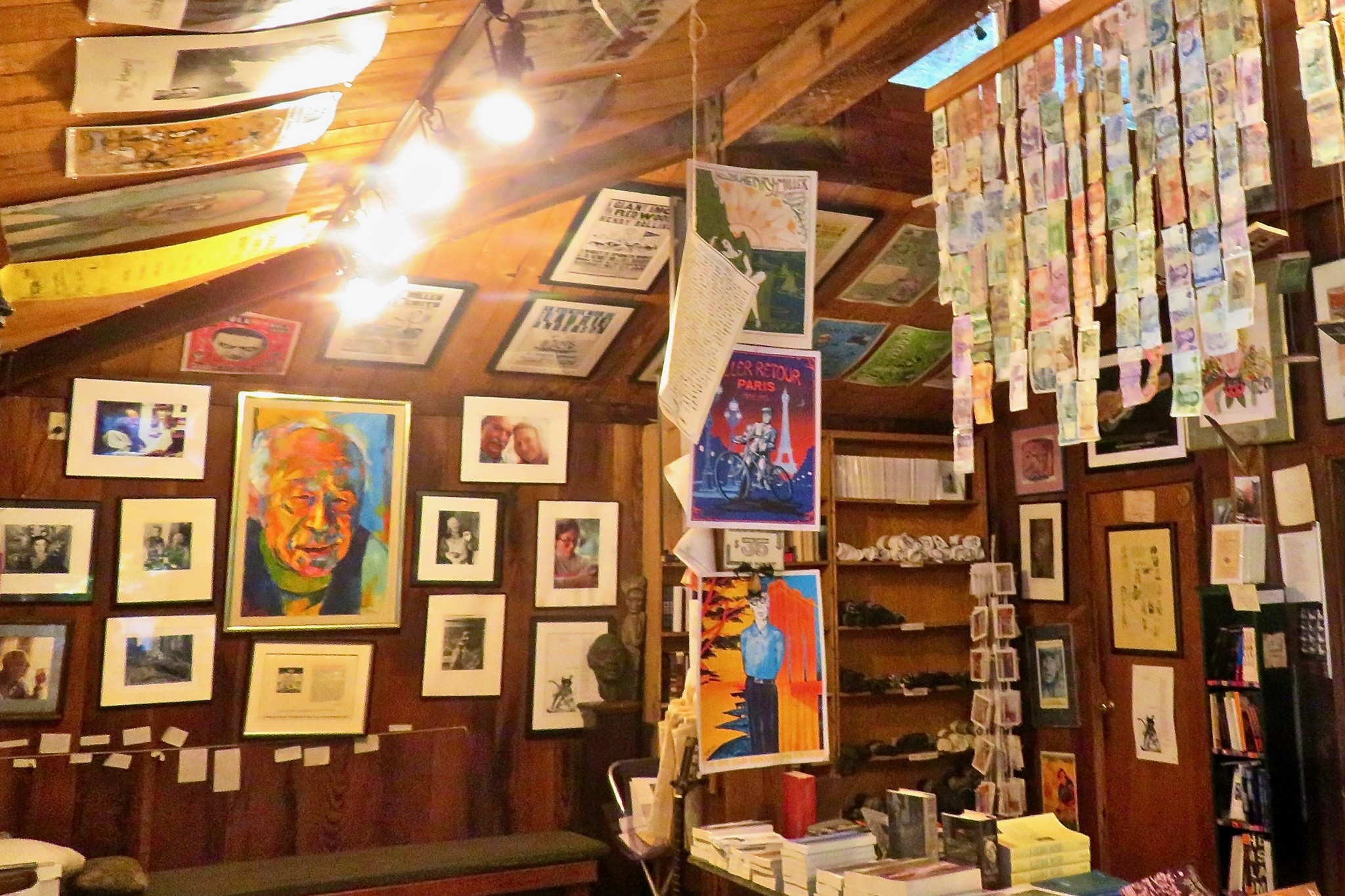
[{"xmin": 74, "ymin": 853, "xmax": 149, "ymax": 893}]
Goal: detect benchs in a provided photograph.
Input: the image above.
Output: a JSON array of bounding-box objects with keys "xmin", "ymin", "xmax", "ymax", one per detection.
[{"xmin": 61, "ymin": 825, "xmax": 617, "ymax": 896}]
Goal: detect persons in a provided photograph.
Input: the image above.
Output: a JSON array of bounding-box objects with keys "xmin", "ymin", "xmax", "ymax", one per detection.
[
  {"xmin": 621, "ymin": 576, "xmax": 647, "ymax": 673},
  {"xmin": 587, "ymin": 633, "xmax": 640, "ymax": 702},
  {"xmin": 554, "ymin": 519, "xmax": 599, "ymax": 589},
  {"xmin": 0, "ymin": 650, "xmax": 43, "ymax": 700},
  {"xmin": 21, "ymin": 525, "xmax": 67, "ymax": 573},
  {"xmin": 479, "ymin": 415, "xmax": 550, "ymax": 466},
  {"xmin": 211, "ymin": 327, "xmax": 266, "ymax": 364}
]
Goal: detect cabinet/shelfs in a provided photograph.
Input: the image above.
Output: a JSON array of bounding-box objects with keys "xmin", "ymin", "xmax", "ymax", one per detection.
[
  {"xmin": 1202, "ymin": 578, "xmax": 1294, "ymax": 896},
  {"xmin": 824, "ymin": 422, "xmax": 982, "ymax": 807}
]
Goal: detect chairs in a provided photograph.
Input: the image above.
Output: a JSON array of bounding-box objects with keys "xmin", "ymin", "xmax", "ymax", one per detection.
[{"xmin": 605, "ymin": 755, "xmax": 680, "ymax": 896}]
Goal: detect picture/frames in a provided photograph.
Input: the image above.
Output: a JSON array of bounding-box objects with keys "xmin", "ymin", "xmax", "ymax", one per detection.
[
  {"xmin": 179, "ymin": 310, "xmax": 302, "ymax": 378},
  {"xmin": 688, "ymin": 156, "xmax": 952, "ymax": 781},
  {"xmin": 1009, "ymin": 421, "xmax": 1089, "ymax": 843},
  {"xmin": 482, "ymin": 288, "xmax": 642, "ymax": 383},
  {"xmin": 1100, "ymin": 520, "xmax": 1188, "ymax": 661},
  {"xmin": 316, "ymin": 277, "xmax": 481, "ymax": 372},
  {"xmin": 0, "ymin": 377, "xmax": 621, "ymax": 746},
  {"xmin": 537, "ymin": 181, "xmax": 689, "ymax": 299}
]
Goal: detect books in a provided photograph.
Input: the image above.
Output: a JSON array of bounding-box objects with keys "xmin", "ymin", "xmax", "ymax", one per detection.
[
  {"xmin": 781, "ymin": 770, "xmax": 817, "ymax": 839},
  {"xmin": 1119, "ymin": 864, "xmax": 1321, "ymax": 896},
  {"xmin": 689, "ymin": 813, "xmax": 1091, "ymax": 896},
  {"xmin": 885, "ymin": 787, "xmax": 940, "ymax": 863},
  {"xmin": 1203, "ymin": 624, "xmax": 1273, "ymax": 896}
]
